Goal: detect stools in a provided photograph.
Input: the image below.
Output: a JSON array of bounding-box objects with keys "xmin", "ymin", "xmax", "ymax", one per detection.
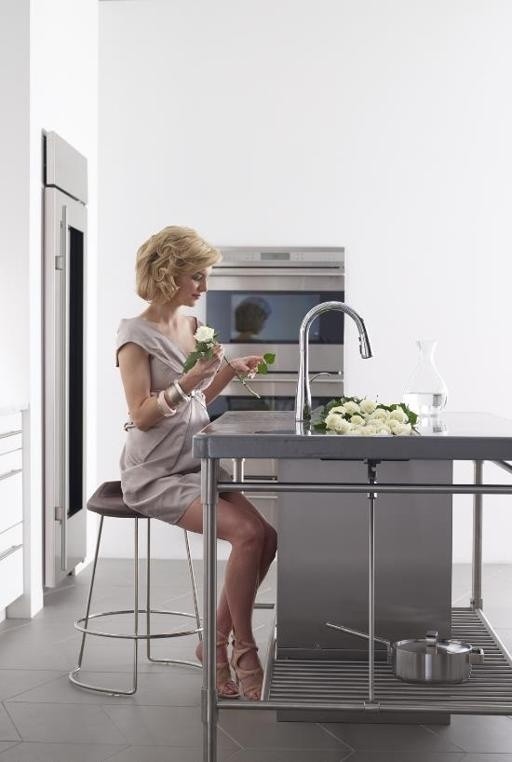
[{"xmin": 67, "ymin": 481, "xmax": 203, "ymax": 696}]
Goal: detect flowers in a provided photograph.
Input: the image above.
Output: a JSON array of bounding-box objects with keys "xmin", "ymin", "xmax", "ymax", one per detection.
[
  {"xmin": 308, "ymin": 397, "xmax": 419, "ymax": 435},
  {"xmin": 183, "ymin": 322, "xmax": 276, "ymax": 399}
]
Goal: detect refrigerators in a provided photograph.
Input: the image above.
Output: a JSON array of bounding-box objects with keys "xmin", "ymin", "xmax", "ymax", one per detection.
[{"xmin": 45, "ymin": 126, "xmax": 92, "ymax": 590}]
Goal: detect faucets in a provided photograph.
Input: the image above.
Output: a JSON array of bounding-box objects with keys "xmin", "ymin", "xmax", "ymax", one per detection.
[{"xmin": 296, "ymin": 300, "xmax": 372, "ymax": 424}]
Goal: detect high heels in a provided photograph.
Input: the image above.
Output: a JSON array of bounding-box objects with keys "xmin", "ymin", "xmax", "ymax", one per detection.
[{"xmin": 193, "ymin": 627, "xmax": 263, "ymax": 701}]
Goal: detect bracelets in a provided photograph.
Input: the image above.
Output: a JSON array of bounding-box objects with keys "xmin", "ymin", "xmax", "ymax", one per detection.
[{"xmin": 156, "ymin": 379, "xmax": 191, "ymax": 418}]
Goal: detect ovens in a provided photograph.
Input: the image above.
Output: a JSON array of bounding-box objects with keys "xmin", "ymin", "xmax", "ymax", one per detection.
[{"xmin": 200, "ymin": 248, "xmax": 343, "ymax": 405}]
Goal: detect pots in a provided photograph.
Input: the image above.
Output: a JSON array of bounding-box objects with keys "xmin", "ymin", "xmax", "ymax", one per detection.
[{"xmin": 327, "ymin": 622, "xmax": 485, "ymax": 685}]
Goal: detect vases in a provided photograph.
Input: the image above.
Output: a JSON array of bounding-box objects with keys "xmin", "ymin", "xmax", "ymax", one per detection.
[{"xmin": 414, "ymin": 415, "xmax": 448, "ymax": 435}]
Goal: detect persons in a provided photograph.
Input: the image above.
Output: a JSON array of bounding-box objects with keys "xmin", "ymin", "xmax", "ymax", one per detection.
[
  {"xmin": 114, "ymin": 226, "xmax": 277, "ymax": 699},
  {"xmin": 233, "ymin": 295, "xmax": 273, "ymax": 341}
]
